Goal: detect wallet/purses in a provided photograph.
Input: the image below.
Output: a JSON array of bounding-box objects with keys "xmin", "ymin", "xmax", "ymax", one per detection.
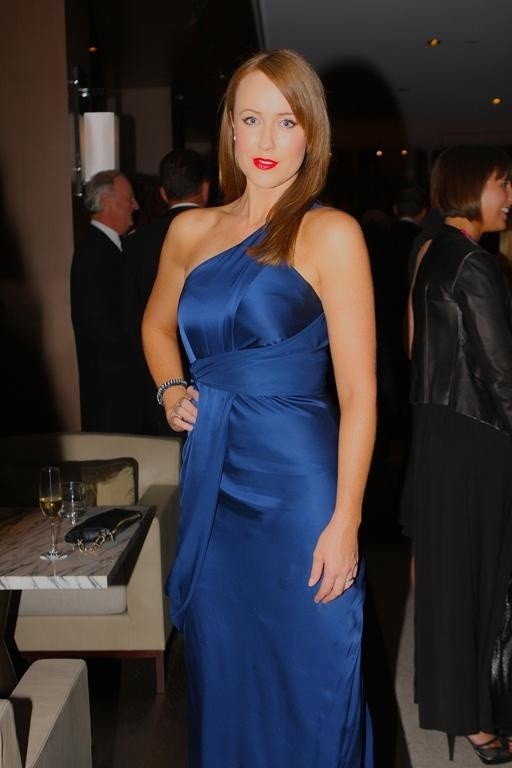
[{"xmin": 65, "ymin": 509, "xmax": 142, "ymax": 543}]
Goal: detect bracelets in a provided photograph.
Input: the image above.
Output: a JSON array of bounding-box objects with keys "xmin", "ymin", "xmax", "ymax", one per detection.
[{"xmin": 156, "ymin": 378, "xmax": 189, "ymax": 407}]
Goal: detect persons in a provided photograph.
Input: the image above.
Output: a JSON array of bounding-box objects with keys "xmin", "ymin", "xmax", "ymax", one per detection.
[
  {"xmin": 138, "ymin": 46, "xmax": 382, "ymax": 763},
  {"xmin": 148, "ymin": 147, "xmax": 208, "ymax": 319},
  {"xmin": 407, "ymin": 143, "xmax": 510, "ymax": 765},
  {"xmin": 384, "ymin": 188, "xmax": 431, "ymax": 407},
  {"xmin": 69, "ymin": 168, "xmax": 145, "ymax": 436}
]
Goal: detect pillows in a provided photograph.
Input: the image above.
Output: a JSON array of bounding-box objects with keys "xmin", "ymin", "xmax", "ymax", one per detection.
[{"xmin": 46, "ymin": 457, "xmax": 138, "ymax": 507}]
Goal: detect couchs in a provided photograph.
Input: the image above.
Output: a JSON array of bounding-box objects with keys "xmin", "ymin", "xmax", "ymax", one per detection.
[
  {"xmin": 0, "ymin": 660, "xmax": 95, "ymax": 768},
  {"xmin": 14, "ymin": 431, "xmax": 186, "ymax": 694}
]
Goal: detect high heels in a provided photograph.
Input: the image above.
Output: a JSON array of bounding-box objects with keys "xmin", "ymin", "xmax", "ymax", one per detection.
[{"xmin": 448, "ymin": 727, "xmax": 512, "ymax": 765}]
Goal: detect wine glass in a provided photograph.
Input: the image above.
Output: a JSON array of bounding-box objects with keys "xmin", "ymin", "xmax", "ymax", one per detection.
[
  {"xmin": 60, "ymin": 480, "xmax": 95, "ymax": 555},
  {"xmin": 36, "ymin": 465, "xmax": 69, "ymax": 562}
]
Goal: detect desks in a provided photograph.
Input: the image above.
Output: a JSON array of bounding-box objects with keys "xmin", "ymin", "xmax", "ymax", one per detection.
[{"xmin": 2, "ymin": 504, "xmax": 152, "ymax": 691}]
